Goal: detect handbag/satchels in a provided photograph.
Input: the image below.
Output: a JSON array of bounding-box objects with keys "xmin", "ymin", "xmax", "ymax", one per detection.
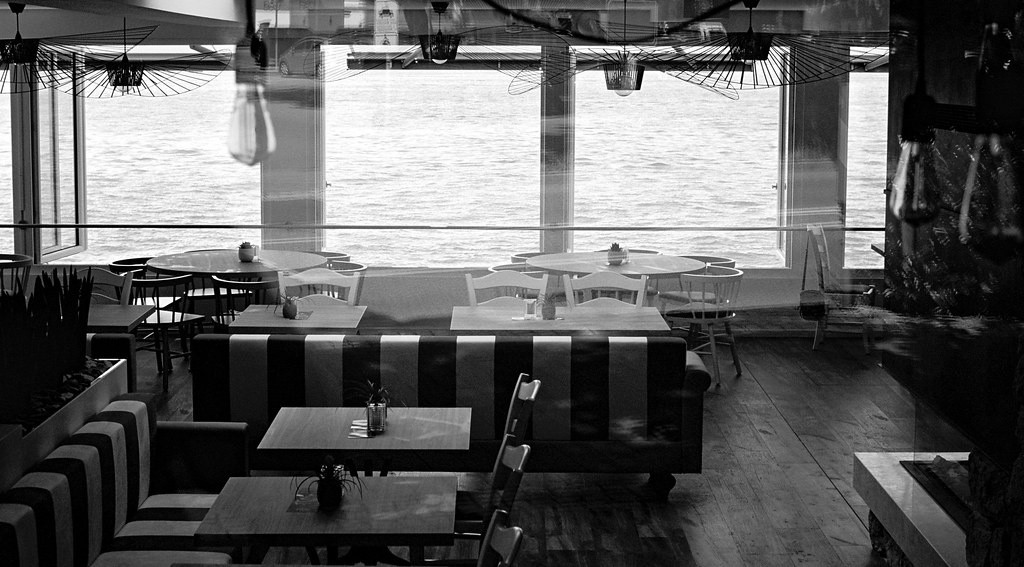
[{"xmin": 800, "ymin": 290, "xmax": 823, "ymax": 319}]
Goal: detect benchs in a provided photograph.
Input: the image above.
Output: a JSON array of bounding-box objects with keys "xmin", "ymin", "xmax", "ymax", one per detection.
[{"xmin": 1, "ymin": 396, "xmax": 252, "ymax": 566}]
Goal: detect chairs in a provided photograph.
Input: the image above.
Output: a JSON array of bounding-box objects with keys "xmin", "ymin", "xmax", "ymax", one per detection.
[
  {"xmin": 1, "ymin": 248, "xmax": 366, "ymax": 392},
  {"xmin": 466, "ymin": 250, "xmax": 743, "ymax": 387},
  {"xmin": 810, "ymin": 224, "xmax": 877, "ymax": 353},
  {"xmin": 384, "ymin": 372, "xmax": 541, "ymax": 567}
]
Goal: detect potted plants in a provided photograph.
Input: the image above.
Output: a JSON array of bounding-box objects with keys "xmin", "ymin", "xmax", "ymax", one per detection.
[
  {"xmin": 0, "ymin": 267, "xmax": 128, "ymax": 493},
  {"xmin": 536, "ymin": 292, "xmax": 557, "ymax": 318},
  {"xmin": 237, "ymin": 241, "xmax": 255, "ymax": 261},
  {"xmin": 608, "ymin": 243, "xmax": 626, "ymax": 265},
  {"xmin": 293, "ymin": 463, "xmax": 370, "ymax": 515},
  {"xmin": 361, "ymin": 379, "xmax": 407, "ymax": 434}
]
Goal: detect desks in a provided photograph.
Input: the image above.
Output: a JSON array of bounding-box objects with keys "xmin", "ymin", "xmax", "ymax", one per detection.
[
  {"xmin": 523, "ymin": 251, "xmax": 706, "ymax": 312},
  {"xmin": 449, "ymin": 305, "xmax": 672, "ymax": 338},
  {"xmin": 193, "ymin": 477, "xmax": 457, "ymax": 567},
  {"xmin": 230, "ymin": 305, "xmax": 369, "ymax": 336},
  {"xmin": 86, "ymin": 304, "xmax": 155, "ymax": 332},
  {"xmin": 149, "ymin": 251, "xmax": 327, "ymax": 313},
  {"xmin": 256, "ymin": 407, "xmax": 473, "ymax": 476}
]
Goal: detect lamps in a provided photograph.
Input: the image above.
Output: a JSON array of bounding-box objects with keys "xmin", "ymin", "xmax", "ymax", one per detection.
[{"xmin": 0, "ymin": 0, "xmax": 906, "ymax": 162}]
